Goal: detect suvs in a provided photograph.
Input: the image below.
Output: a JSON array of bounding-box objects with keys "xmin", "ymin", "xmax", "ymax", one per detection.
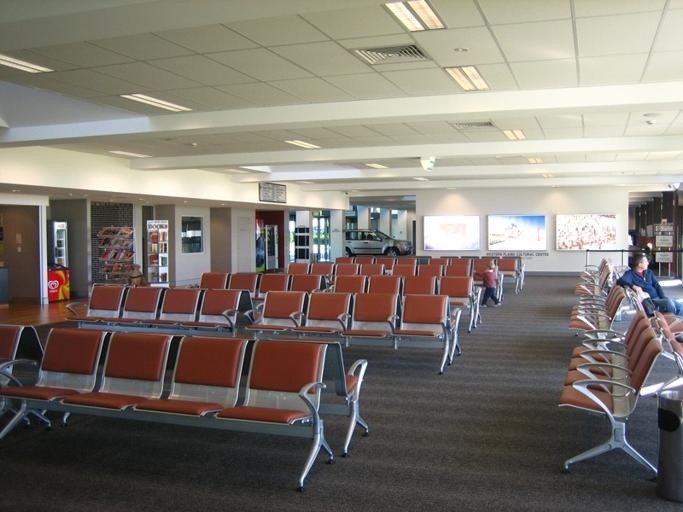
[{"xmin": 343, "ymin": 229, "xmax": 410, "ymax": 256}]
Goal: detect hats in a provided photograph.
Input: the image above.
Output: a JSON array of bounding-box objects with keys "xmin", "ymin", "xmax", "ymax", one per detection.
[{"xmin": 487, "ymin": 263, "xmax": 495, "ymax": 269}]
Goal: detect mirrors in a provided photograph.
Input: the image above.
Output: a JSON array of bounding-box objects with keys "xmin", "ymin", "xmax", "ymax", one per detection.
[{"xmin": 182, "ymin": 216, "xmax": 203, "ymax": 253}]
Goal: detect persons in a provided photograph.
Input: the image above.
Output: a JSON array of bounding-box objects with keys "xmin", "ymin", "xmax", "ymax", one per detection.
[
  {"xmin": 472, "ymin": 262, "xmax": 501, "ymax": 306},
  {"xmin": 615, "ymin": 254, "xmax": 682, "ymax": 314}
]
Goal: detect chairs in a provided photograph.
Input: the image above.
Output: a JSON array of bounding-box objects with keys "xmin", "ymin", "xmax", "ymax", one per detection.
[
  {"xmin": 576, "ymin": 270, "xmax": 611, "ymax": 295},
  {"xmin": 336, "ymin": 258, "xmax": 353, "ymax": 264},
  {"xmin": 392, "ymin": 264, "xmax": 415, "ymax": 278},
  {"xmin": 402, "ymin": 276, "xmax": 436, "ymax": 294},
  {"xmin": 0, "ymin": 325, "xmax": 30, "ymax": 425},
  {"xmin": 360, "ymin": 264, "xmax": 384, "ymax": 277},
  {"xmin": 418, "ymin": 265, "xmax": 442, "ymax": 280},
  {"xmin": 472, "ymin": 259, "xmax": 504, "ymax": 302},
  {"xmin": 656, "ymin": 318, "xmax": 670, "ymax": 332},
  {"xmin": 399, "ymin": 259, "xmax": 417, "ymax": 265},
  {"xmin": 244, "ymin": 291, "xmax": 306, "ymax": 339},
  {"xmin": 461, "ymin": 256, "xmax": 479, "ymax": 270},
  {"xmin": 581, "ymin": 261, "xmax": 607, "ymax": 283},
  {"xmin": 625, "ymin": 285, "xmax": 675, "ymax": 315},
  {"xmin": 335, "ymin": 263, "xmax": 359, "ymax": 275},
  {"xmin": 568, "ymin": 318, "xmax": 649, "ymax": 376},
  {"xmin": 628, "ymin": 288, "xmax": 680, "ymax": 327},
  {"xmin": 290, "ymin": 275, "xmax": 321, "ymax": 294},
  {"xmin": 287, "ymin": 263, "xmax": 310, "ymax": 274},
  {"xmin": 236, "ymin": 290, "xmax": 264, "ymax": 328},
  {"xmin": 482, "ymin": 257, "xmax": 500, "ymax": 266},
  {"xmin": 133, "ymin": 336, "xmax": 248, "ymax": 417},
  {"xmin": 228, "ymin": 273, "xmax": 258, "ymax": 298},
  {"xmin": 258, "ymin": 274, "xmax": 291, "ymax": 299},
  {"xmin": 654, "ymin": 311, "xmax": 666, "ymax": 322},
  {"xmin": 558, "ymin": 337, "xmax": 662, "ymax": 478},
  {"xmin": 496, "ymin": 259, "xmax": 520, "ymax": 294},
  {"xmin": 200, "ymin": 272, "xmax": 230, "ymax": 289},
  {"xmin": 399, "ymin": 256, "xmax": 415, "ymax": 258},
  {"xmin": 355, "ymin": 257, "xmax": 374, "ymax": 264},
  {"xmin": 564, "ymin": 326, "xmax": 655, "ymax": 392},
  {"xmin": 581, "ymin": 257, "xmax": 605, "ymax": 274},
  {"xmin": 379, "ymin": 256, "xmax": 398, "ymax": 264},
  {"xmin": 660, "ymin": 328, "xmax": 676, "ymax": 342},
  {"xmin": 139, "ymin": 289, "xmax": 201, "ymax": 335},
  {"xmin": 430, "ymin": 258, "xmax": 447, "ymax": 275},
  {"xmin": 576, "ymin": 269, "xmax": 609, "ymax": 292},
  {"xmin": 320, "ymin": 276, "xmax": 334, "ymax": 293},
  {"xmin": 656, "ymin": 339, "xmax": 683, "ymax": 396},
  {"xmin": 322, "ymin": 276, "xmax": 366, "ymax": 296},
  {"xmin": 571, "ymin": 310, "xmax": 645, "ymax": 362},
  {"xmin": 576, "ymin": 266, "xmax": 608, "ymax": 289},
  {"xmin": 367, "ymin": 276, "xmax": 400, "ymax": 293},
  {"xmin": 440, "ymin": 257, "xmax": 459, "ymax": 265},
  {"xmin": 101, "ymin": 288, "xmax": 163, "ymax": 328},
  {"xmin": 631, "ymin": 292, "xmax": 683, "ymax": 361},
  {"xmin": 60, "ymin": 332, "xmax": 173, "ymax": 412},
  {"xmin": 0, "ymin": 328, "xmax": 107, "ymax": 438},
  {"xmin": 576, "ymin": 264, "xmax": 607, "ymax": 287},
  {"xmin": 571, "ymin": 285, "xmax": 620, "ymax": 315},
  {"xmin": 568, "ymin": 292, "xmax": 626, "ymax": 330},
  {"xmin": 450, "ymin": 258, "xmax": 471, "ymax": 266},
  {"xmin": 66, "ymin": 286, "xmax": 126, "ymax": 327},
  {"xmin": 470, "ymin": 277, "xmax": 482, "ymax": 328},
  {"xmin": 341, "ymin": 294, "xmax": 398, "ymax": 350},
  {"xmin": 571, "ymin": 288, "xmax": 623, "ymax": 320},
  {"xmin": 311, "ymin": 263, "xmax": 334, "ymax": 281},
  {"xmin": 15, "ymin": 326, "xmax": 48, "ymax": 415},
  {"xmin": 572, "ymin": 284, "xmax": 617, "ymax": 338},
  {"xmin": 376, "ymin": 258, "xmax": 394, "ymax": 269},
  {"xmin": 445, "ymin": 265, "xmax": 469, "ymax": 276},
  {"xmin": 291, "ymin": 292, "xmax": 351, "ymax": 348},
  {"xmin": 503, "ymin": 256, "xmax": 525, "ymax": 291},
  {"xmin": 391, "ymin": 294, "xmax": 450, "ymax": 375},
  {"xmin": 289, "ymin": 340, "xmax": 368, "ymax": 458},
  {"xmin": 416, "ymin": 256, "xmax": 432, "ymax": 264},
  {"xmin": 215, "ymin": 340, "xmax": 333, "ymax": 493},
  {"xmin": 440, "ymin": 277, "xmax": 476, "ymax": 333},
  {"xmin": 358, "ymin": 255, "xmax": 377, "ymax": 264},
  {"xmin": 181, "ymin": 290, "xmax": 242, "ymax": 338}
]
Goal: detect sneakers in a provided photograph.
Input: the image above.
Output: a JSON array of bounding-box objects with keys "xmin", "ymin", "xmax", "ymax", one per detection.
[
  {"xmin": 492, "ymin": 303, "xmax": 502, "ymax": 308},
  {"xmin": 675, "ymin": 332, "xmax": 683, "ymax": 343},
  {"xmin": 479, "ymin": 304, "xmax": 487, "ymax": 308}
]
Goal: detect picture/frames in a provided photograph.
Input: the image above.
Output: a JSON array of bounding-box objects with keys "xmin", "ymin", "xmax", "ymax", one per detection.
[
  {"xmin": 555, "ymin": 214, "xmax": 617, "ymax": 251},
  {"xmin": 487, "ymin": 214, "xmax": 546, "ymax": 251},
  {"xmin": 423, "ymin": 215, "xmax": 480, "ymax": 251},
  {"xmin": 259, "ymin": 182, "xmax": 286, "ymax": 203}
]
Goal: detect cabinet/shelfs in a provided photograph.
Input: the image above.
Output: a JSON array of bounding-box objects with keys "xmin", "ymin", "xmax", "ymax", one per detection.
[
  {"xmin": 96, "ymin": 225, "xmax": 136, "ymax": 283},
  {"xmin": 47, "ymin": 220, "xmax": 68, "ymax": 267},
  {"xmin": 147, "ymin": 220, "xmax": 169, "ymax": 283}
]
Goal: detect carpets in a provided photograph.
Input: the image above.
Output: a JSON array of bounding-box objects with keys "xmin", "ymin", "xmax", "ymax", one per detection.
[
  {"xmin": 0, "ymin": 276, "xmax": 683, "ymax": 512},
  {"xmin": 649, "ymin": 266, "xmax": 671, "ymax": 276}
]
